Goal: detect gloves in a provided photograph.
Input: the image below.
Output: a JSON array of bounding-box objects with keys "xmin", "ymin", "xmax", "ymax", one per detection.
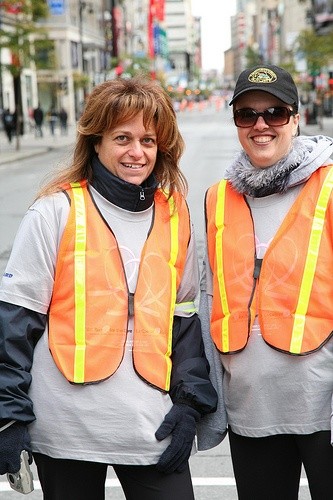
[
  {"xmin": 0, "ymin": 418, "xmax": 33, "ymax": 476},
  {"xmin": 155, "ymin": 402, "xmax": 201, "ymax": 476}
]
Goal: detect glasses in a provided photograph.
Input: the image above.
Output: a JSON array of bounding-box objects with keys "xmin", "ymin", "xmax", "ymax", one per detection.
[{"xmin": 232, "ymin": 106, "xmax": 298, "ymax": 127}]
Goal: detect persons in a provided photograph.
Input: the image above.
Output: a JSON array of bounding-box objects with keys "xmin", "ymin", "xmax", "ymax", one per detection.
[
  {"xmin": 1, "ymin": 79, "xmax": 218, "ymax": 500},
  {"xmin": 199, "ymin": 63, "xmax": 332, "ymax": 500},
  {"xmin": 2, "ymin": 99, "xmax": 71, "ymax": 146}
]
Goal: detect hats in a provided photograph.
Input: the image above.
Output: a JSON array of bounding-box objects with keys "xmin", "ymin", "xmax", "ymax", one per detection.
[{"xmin": 228, "ymin": 65, "xmax": 298, "ymax": 106}]
